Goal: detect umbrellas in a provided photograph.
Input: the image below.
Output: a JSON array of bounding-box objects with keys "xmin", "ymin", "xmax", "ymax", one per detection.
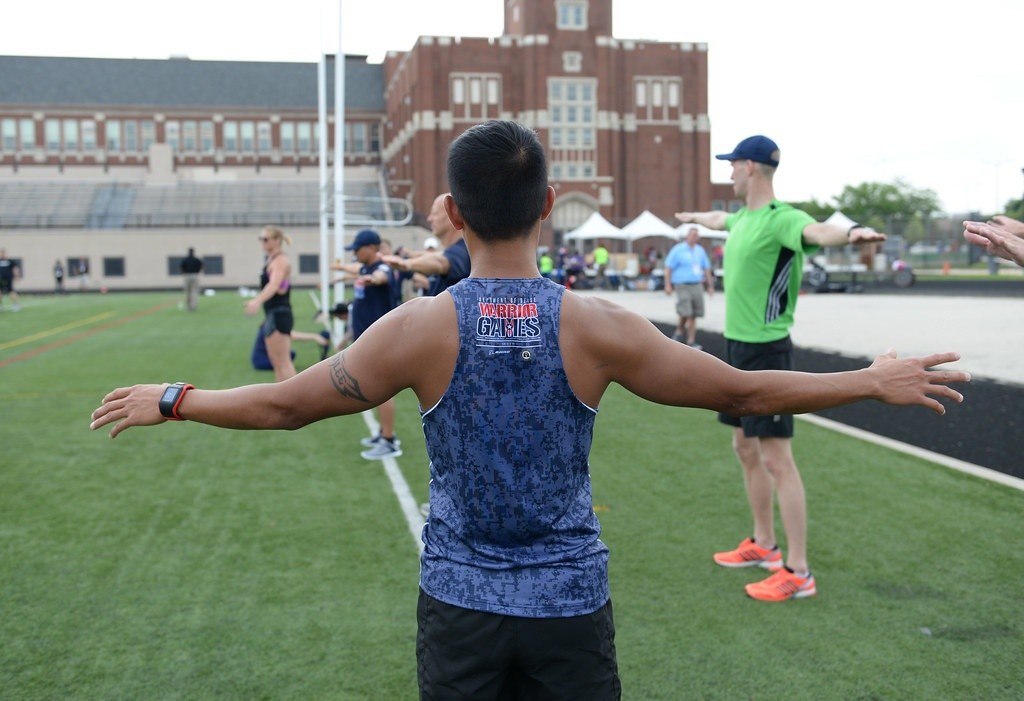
[
  {"xmin": 617, "ymin": 211, "xmax": 678, "ymax": 259},
  {"xmin": 814, "ymin": 211, "xmax": 879, "ymax": 263},
  {"xmin": 570, "ymin": 211, "xmax": 626, "ymax": 260},
  {"xmin": 669, "ymin": 214, "xmax": 731, "ymax": 246}
]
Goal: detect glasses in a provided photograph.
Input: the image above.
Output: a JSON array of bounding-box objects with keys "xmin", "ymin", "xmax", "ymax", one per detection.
[{"xmin": 258, "ymin": 237, "xmax": 272, "ymax": 243}]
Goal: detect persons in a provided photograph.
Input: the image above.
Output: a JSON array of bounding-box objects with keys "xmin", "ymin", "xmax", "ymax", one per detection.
[
  {"xmin": 960, "ymin": 213, "xmax": 1024, "ymax": 272},
  {"xmin": 90, "ymin": 119, "xmax": 971, "ymax": 701},
  {"xmin": 242, "ymin": 222, "xmax": 713, "ymax": 461},
  {"xmin": 0, "ymin": 250, "xmax": 25, "ymax": 312},
  {"xmin": 55, "ymin": 260, "xmax": 66, "ymax": 296},
  {"xmin": 678, "ymin": 133, "xmax": 886, "ymax": 605},
  {"xmin": 181, "ymin": 246, "xmax": 203, "ymax": 316},
  {"xmin": 76, "ymin": 255, "xmax": 88, "ymax": 294}
]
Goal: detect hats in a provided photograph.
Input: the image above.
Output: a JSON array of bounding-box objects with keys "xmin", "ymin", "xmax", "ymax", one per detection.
[
  {"xmin": 716, "ymin": 135, "xmax": 779, "ymax": 167},
  {"xmin": 343, "ymin": 230, "xmax": 380, "ymax": 249},
  {"xmin": 423, "ymin": 238, "xmax": 438, "ymax": 250}
]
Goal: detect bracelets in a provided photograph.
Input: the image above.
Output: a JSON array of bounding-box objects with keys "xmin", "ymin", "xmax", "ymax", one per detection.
[{"xmin": 847, "ymin": 223, "xmax": 865, "ymax": 237}]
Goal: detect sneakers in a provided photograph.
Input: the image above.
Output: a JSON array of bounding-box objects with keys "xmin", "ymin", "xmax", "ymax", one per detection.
[
  {"xmin": 743, "ymin": 565, "xmax": 817, "ymax": 601},
  {"xmin": 713, "ymin": 536, "xmax": 784, "ymax": 569},
  {"xmin": 361, "ymin": 439, "xmax": 402, "ymax": 460},
  {"xmin": 361, "ymin": 431, "xmax": 401, "ymax": 446}
]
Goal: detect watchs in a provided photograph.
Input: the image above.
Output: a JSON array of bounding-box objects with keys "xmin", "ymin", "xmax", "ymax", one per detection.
[{"xmin": 158, "ymin": 381, "xmax": 196, "ymax": 421}]
